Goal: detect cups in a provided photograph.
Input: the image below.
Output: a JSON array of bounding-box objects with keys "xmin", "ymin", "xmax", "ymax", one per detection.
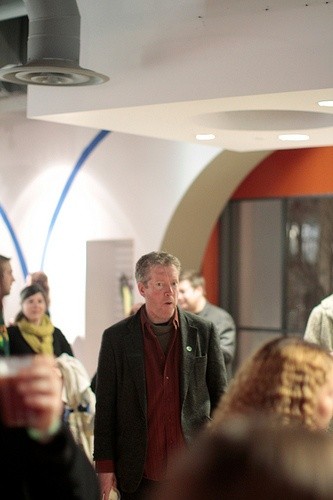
[{"xmin": 0, "ymin": 354, "xmax": 47, "ymax": 427}]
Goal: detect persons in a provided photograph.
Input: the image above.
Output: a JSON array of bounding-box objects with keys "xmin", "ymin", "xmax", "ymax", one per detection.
[
  {"xmin": 0, "ymin": 355, "xmax": 102, "ymax": 500},
  {"xmin": 210, "ymin": 335, "xmax": 333, "ymax": 433},
  {"xmin": 3, "ymin": 283, "xmax": 74, "ymax": 360},
  {"xmin": 302, "ymin": 294, "xmax": 332, "ymax": 356},
  {"xmin": 23, "ymin": 271, "xmax": 49, "ymax": 314},
  {"xmin": 1, "ymin": 254, "xmax": 15, "ymax": 356},
  {"xmin": 174, "ymin": 265, "xmax": 236, "ymax": 385},
  {"xmin": 92, "ymin": 251, "xmax": 229, "ymax": 499}
]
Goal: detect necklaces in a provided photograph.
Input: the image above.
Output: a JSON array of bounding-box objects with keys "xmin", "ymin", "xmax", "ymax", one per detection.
[{"xmin": 155, "ymin": 326, "xmax": 174, "ymax": 337}]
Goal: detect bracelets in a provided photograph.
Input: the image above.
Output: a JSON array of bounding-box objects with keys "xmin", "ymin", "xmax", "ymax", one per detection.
[{"xmin": 26, "ymin": 416, "xmax": 62, "ymax": 441}]
